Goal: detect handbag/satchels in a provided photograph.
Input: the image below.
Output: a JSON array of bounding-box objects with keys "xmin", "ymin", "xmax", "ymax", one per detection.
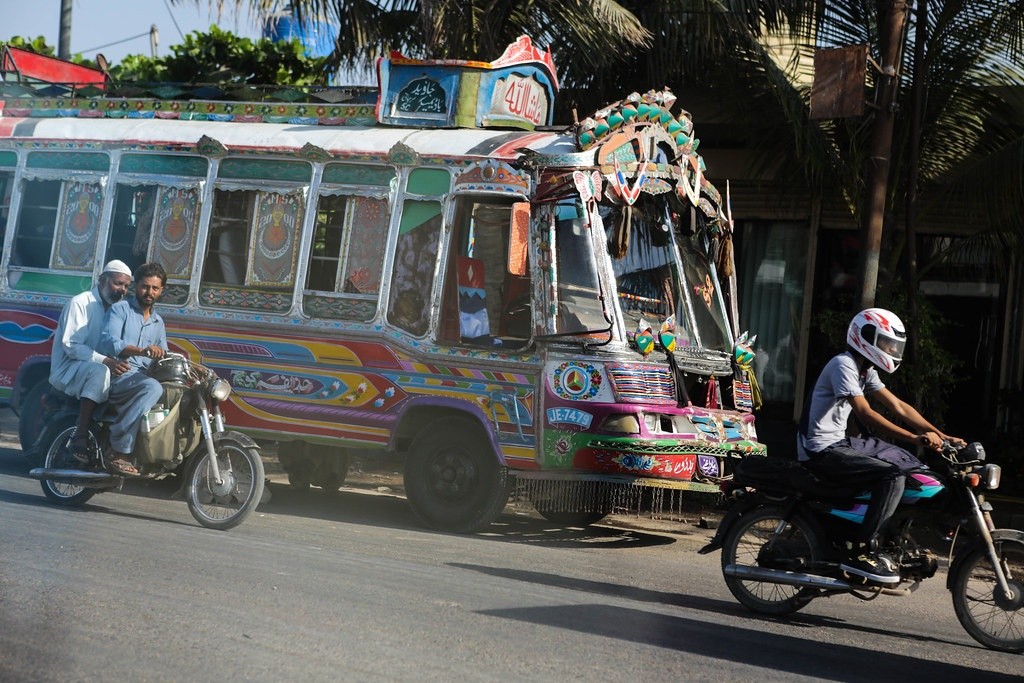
[{"xmin": 133, "ymin": 391, "xmax": 185, "ymax": 470}]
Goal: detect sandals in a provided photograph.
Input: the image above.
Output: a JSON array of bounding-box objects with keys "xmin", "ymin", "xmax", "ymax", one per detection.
[
  {"xmin": 103, "ymin": 450, "xmax": 141, "ymax": 478},
  {"xmin": 69, "ymin": 435, "xmax": 90, "ymax": 461}
]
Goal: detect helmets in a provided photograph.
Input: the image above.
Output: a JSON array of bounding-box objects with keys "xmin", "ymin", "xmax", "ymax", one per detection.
[{"xmin": 846, "ymin": 307, "xmax": 906, "ymax": 373}]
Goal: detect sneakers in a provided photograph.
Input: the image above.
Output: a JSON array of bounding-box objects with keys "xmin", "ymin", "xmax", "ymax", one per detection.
[{"xmin": 840, "ymin": 548, "xmax": 899, "ymax": 584}]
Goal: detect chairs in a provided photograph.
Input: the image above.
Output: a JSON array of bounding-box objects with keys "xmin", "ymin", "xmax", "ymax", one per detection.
[{"xmin": 218, "ymin": 230, "xmax": 248, "ymax": 284}]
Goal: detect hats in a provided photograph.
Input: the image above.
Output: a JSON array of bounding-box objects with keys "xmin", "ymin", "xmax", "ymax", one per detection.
[{"xmin": 105, "ymin": 259, "xmax": 132, "ymax": 277}]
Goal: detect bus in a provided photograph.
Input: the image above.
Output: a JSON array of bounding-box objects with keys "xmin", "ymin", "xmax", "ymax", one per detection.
[{"xmin": 0, "ymin": 32, "xmax": 768, "ymax": 535}]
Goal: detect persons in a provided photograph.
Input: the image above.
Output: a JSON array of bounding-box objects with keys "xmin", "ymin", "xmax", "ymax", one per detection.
[
  {"xmin": 798, "ymin": 308, "xmax": 968, "ymax": 583},
  {"xmin": 98, "ymin": 261, "xmax": 208, "ymax": 475},
  {"xmin": 48, "ymin": 260, "xmax": 133, "ymax": 464},
  {"xmin": 323, "ymin": 211, "xmax": 344, "ymax": 274}
]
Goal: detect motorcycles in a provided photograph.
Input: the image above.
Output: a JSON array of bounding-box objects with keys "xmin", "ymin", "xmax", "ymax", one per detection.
[
  {"xmin": 696, "ymin": 438, "xmax": 1024, "ymax": 653},
  {"xmin": 27, "ymin": 350, "xmax": 265, "ymax": 532}
]
